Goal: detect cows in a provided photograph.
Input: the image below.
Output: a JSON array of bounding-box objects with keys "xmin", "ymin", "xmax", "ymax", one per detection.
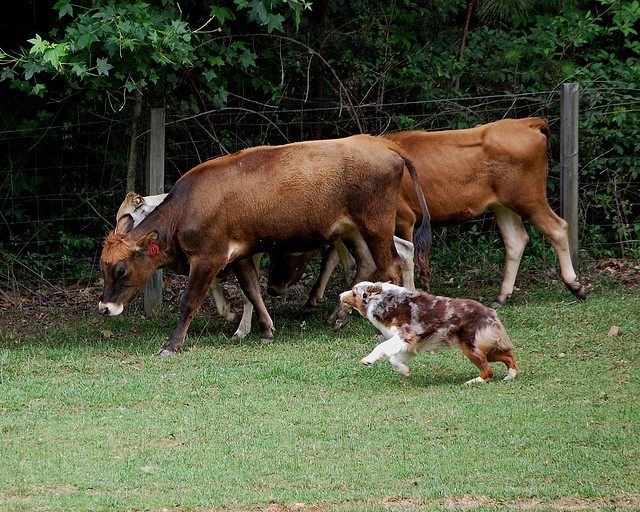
[
  {"xmin": 266, "ymin": 115, "xmax": 587, "ymax": 319},
  {"xmin": 98, "ymin": 132, "xmax": 433, "ymax": 360},
  {"xmin": 113, "ymin": 189, "xmax": 417, "ymax": 344}
]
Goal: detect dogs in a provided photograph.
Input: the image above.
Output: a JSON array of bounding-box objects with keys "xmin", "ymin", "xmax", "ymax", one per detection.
[{"xmin": 338, "ymin": 279, "xmax": 519, "ymax": 386}]
